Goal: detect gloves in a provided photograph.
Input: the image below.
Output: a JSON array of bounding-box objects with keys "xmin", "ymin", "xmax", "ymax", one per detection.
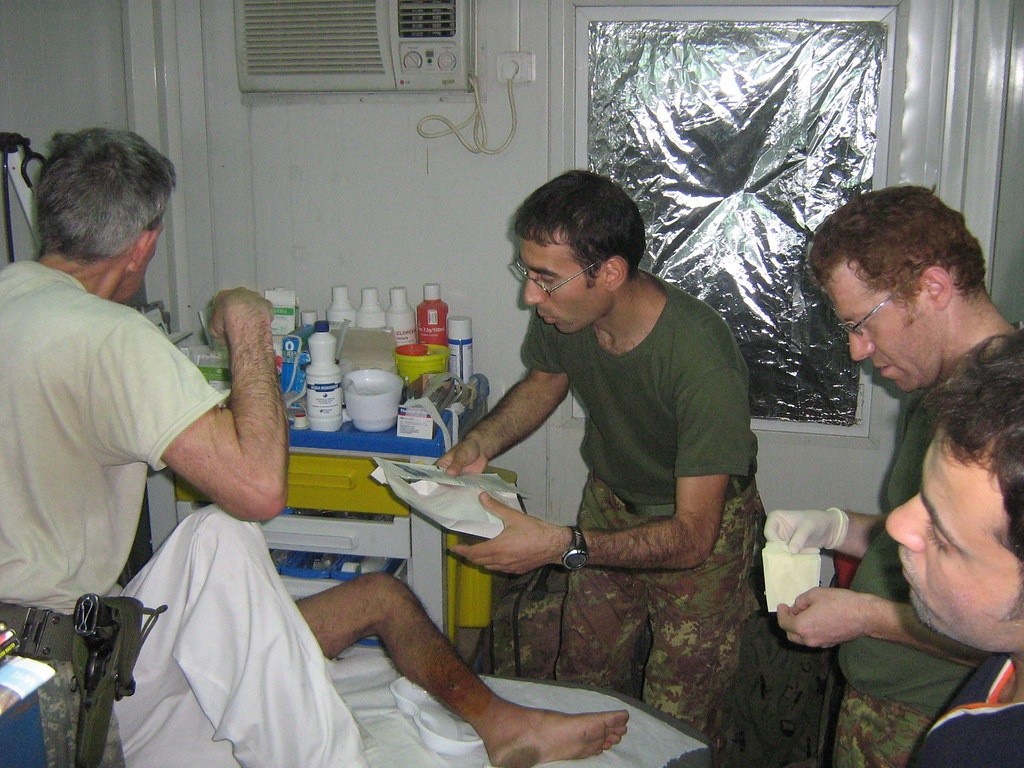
[
  {"xmin": 762, "ymin": 503, "xmax": 849, "ymax": 557},
  {"xmin": 761, "ymin": 540, "xmax": 822, "ymax": 613}
]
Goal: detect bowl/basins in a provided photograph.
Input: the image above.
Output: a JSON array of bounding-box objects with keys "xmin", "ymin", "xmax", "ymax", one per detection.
[
  {"xmin": 396, "ymin": 345, "xmax": 449, "ymax": 387},
  {"xmin": 343, "ymin": 369, "xmax": 403, "ymax": 432}
]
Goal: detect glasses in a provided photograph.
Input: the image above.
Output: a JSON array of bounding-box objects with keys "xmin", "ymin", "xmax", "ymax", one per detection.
[
  {"xmin": 837, "ymin": 273, "xmax": 924, "ymax": 337},
  {"xmin": 512, "ymin": 254, "xmax": 603, "ymax": 297}
]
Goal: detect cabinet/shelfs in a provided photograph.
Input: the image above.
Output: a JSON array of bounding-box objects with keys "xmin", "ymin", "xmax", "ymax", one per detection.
[{"xmin": 146, "ymin": 375, "xmax": 492, "ymax": 657}]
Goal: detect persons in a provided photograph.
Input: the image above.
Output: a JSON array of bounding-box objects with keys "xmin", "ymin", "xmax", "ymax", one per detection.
[
  {"xmin": 0, "ymin": 127, "xmax": 630, "ymax": 768},
  {"xmin": 432, "ymin": 169, "xmax": 763, "ymax": 747},
  {"xmin": 885, "ymin": 324, "xmax": 1024, "ymax": 768},
  {"xmin": 764, "ymin": 186, "xmax": 1024, "ymax": 768}
]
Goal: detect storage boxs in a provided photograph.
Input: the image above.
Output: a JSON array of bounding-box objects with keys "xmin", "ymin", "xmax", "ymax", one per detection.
[
  {"xmin": 198, "ymin": 354, "xmax": 232, "ymax": 407},
  {"xmin": 397, "ymin": 405, "xmax": 434, "ymax": 440},
  {"xmin": 264, "ymin": 289, "xmax": 299, "ymax": 337},
  {"xmin": 459, "ymin": 464, "xmax": 517, "ymax": 631}
]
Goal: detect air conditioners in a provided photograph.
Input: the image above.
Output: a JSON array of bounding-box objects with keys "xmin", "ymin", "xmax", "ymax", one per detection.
[{"xmin": 233, "ymin": 0, "xmax": 476, "ymax": 92}]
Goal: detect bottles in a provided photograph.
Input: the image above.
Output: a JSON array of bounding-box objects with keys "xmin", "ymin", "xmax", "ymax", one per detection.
[
  {"xmin": 327, "ymin": 286, "xmax": 356, "ymax": 338},
  {"xmin": 356, "ymin": 287, "xmax": 386, "ymax": 328},
  {"xmin": 417, "ymin": 282, "xmax": 450, "ymax": 346},
  {"xmin": 306, "ymin": 320, "xmax": 342, "ymax": 432},
  {"xmin": 385, "ymin": 286, "xmax": 416, "ymax": 346}
]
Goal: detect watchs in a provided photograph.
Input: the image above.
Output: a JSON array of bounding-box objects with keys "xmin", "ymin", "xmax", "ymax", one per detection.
[{"xmin": 561, "ymin": 526, "xmax": 589, "ymax": 571}]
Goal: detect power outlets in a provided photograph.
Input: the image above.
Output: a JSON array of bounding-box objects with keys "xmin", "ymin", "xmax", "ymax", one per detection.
[{"xmin": 496, "ymin": 51, "xmax": 536, "ymax": 83}]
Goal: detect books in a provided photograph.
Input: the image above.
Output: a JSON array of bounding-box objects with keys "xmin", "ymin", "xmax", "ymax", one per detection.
[{"xmin": 0, "ymin": 619, "xmax": 55, "ymax": 715}]
